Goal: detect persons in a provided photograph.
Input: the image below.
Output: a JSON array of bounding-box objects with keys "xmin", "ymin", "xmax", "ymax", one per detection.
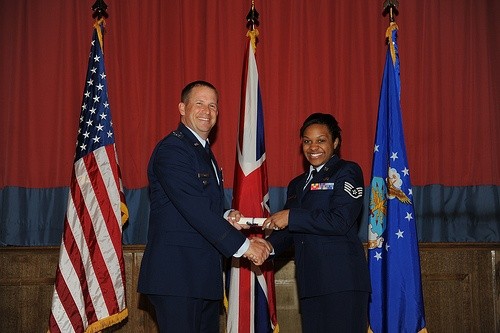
[
  {"xmin": 136, "ymin": 82, "xmax": 275, "ymax": 333},
  {"xmin": 240, "ymin": 112, "xmax": 373, "ymax": 333}
]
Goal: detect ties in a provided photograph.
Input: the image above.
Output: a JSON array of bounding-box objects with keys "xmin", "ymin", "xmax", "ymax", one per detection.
[
  {"xmin": 310, "ymin": 169, "xmax": 318, "ymax": 178},
  {"xmin": 205, "ymin": 141, "xmax": 211, "ymax": 162}
]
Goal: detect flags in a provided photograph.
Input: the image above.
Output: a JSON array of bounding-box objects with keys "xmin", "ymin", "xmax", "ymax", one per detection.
[
  {"xmin": 45, "ymin": 17, "xmax": 130, "ymax": 333},
  {"xmin": 364, "ymin": 22, "xmax": 428, "ymax": 333},
  {"xmin": 223, "ymin": 24, "xmax": 280, "ymax": 333}
]
